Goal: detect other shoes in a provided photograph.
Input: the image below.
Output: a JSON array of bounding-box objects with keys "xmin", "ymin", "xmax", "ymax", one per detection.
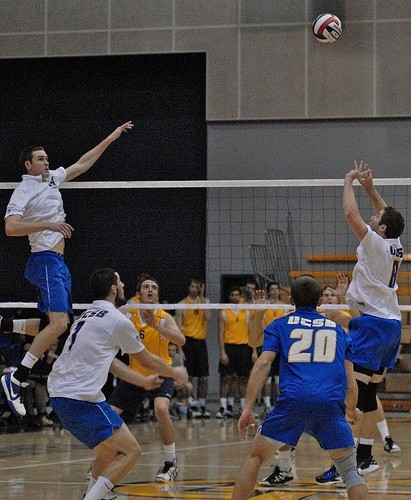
[
  {"xmin": 199, "ymin": 406, "xmax": 211, "ymax": 417},
  {"xmin": 81, "ymin": 488, "xmax": 129, "ymax": 500},
  {"xmin": 216, "ymin": 405, "xmax": 235, "ymax": 418},
  {"xmin": 261, "ymin": 407, "xmax": 271, "ymax": 419},
  {"xmin": 186, "ymin": 403, "xmax": 202, "ymax": 418},
  {"xmin": 42, "ymin": 416, "xmax": 54, "ymax": 426}
]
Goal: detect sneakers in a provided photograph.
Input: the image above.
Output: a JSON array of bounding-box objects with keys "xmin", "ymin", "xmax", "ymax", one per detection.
[
  {"xmin": 1, "ymin": 371, "xmax": 29, "ymax": 417},
  {"xmin": 314, "ymin": 464, "xmax": 343, "ymax": 486},
  {"xmin": 258, "ymin": 464, "xmax": 296, "ymax": 486},
  {"xmin": 384, "ymin": 436, "xmax": 402, "ymax": 453},
  {"xmin": 340, "ymin": 454, "xmax": 380, "ymax": 483},
  {"xmin": 154, "ymin": 457, "xmax": 179, "ymax": 482}
]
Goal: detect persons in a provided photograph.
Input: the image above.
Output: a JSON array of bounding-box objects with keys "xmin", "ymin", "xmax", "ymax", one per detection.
[
  {"xmin": 85, "ymin": 276, "xmax": 186, "ymax": 482},
  {"xmin": 1, "ymin": 120, "xmax": 134, "ymax": 417},
  {"xmin": 232, "ymin": 276, "xmax": 369, "ymax": 500},
  {"xmin": 47, "ymin": 268, "xmax": 189, "ymax": 500},
  {"xmin": 216, "ymin": 272, "xmax": 401, "ymax": 488},
  {"xmin": 315, "ymin": 159, "xmax": 405, "ymax": 486},
  {"xmin": 0, "ymin": 292, "xmax": 192, "ymax": 429},
  {"xmin": 176, "ymin": 278, "xmax": 211, "ymax": 419}
]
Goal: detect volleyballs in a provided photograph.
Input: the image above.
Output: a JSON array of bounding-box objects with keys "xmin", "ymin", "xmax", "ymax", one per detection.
[{"xmin": 312, "ymin": 12, "xmax": 342, "ymax": 43}]
